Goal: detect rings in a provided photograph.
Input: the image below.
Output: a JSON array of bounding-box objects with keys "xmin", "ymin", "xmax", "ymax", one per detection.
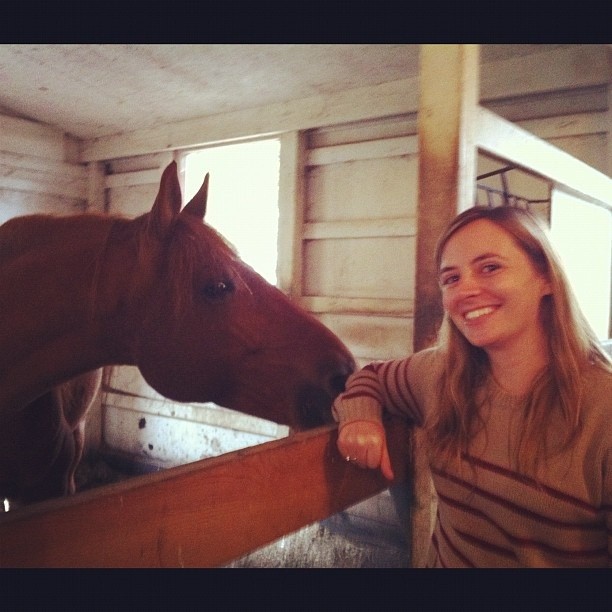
[{"xmin": 343, "ymin": 455, "xmax": 358, "ymax": 463}]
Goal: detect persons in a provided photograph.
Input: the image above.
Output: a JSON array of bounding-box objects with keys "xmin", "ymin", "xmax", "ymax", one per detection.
[{"xmin": 331, "ymin": 205, "xmax": 612, "ymax": 568}]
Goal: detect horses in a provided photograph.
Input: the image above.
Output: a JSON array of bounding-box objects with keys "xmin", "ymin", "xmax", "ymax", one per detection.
[{"xmin": 0, "ymin": 160, "xmax": 362, "ymax": 515}]
